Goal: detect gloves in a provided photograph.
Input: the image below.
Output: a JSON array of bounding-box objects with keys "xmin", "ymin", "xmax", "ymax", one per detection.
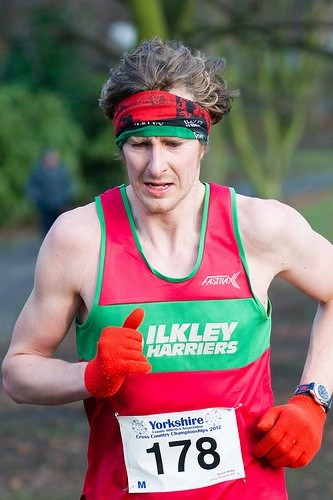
[
  {"xmin": 85, "ymin": 308, "xmax": 151, "ymax": 399},
  {"xmin": 252, "ymin": 395, "xmax": 327, "ymax": 469}
]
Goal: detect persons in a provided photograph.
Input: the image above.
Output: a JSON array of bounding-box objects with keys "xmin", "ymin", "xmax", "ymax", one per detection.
[
  {"xmin": 25, "ymin": 146, "xmax": 73, "ymax": 243},
  {"xmin": 0, "ymin": 36, "xmax": 332, "ymax": 500}
]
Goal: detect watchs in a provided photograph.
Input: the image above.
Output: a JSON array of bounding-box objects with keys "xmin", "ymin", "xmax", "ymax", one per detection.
[{"xmin": 292, "ymin": 381, "xmax": 331, "ymax": 415}]
